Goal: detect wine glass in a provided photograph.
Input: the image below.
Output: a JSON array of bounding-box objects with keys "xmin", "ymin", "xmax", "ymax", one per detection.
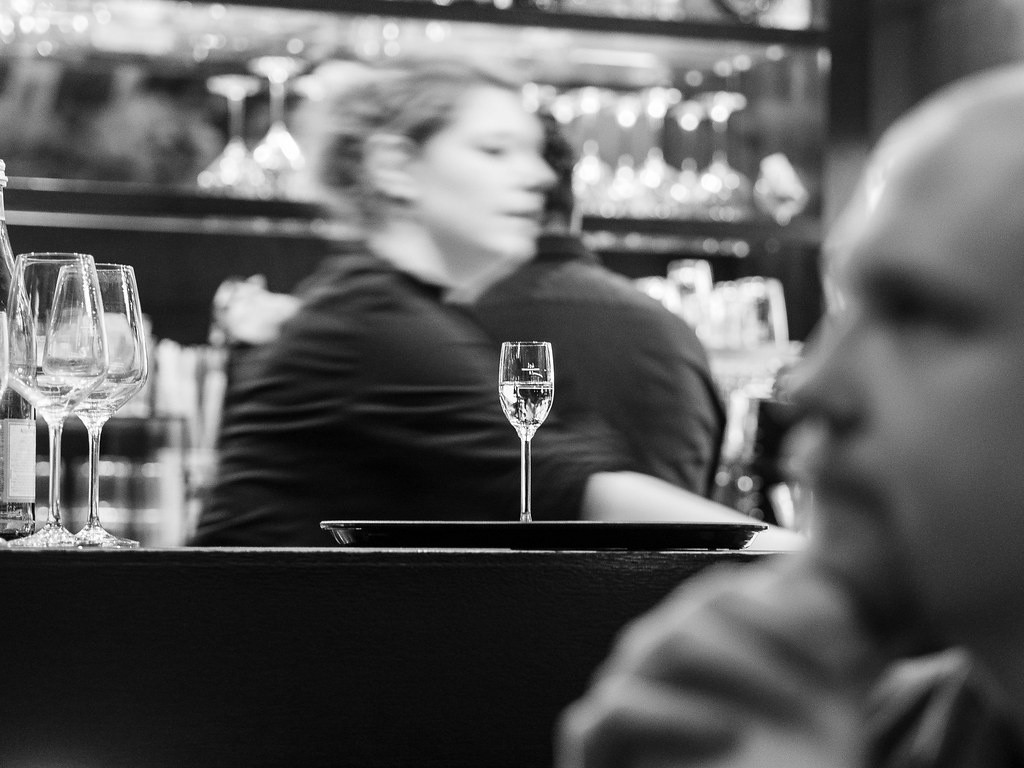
[
  {"xmin": 40, "ymin": 259, "xmax": 147, "ymax": 552},
  {"xmin": 196, "ymin": 53, "xmax": 810, "ymax": 225},
  {"xmin": 4, "ymin": 251, "xmax": 109, "ymax": 549},
  {"xmin": 498, "ymin": 341, "xmax": 555, "ymax": 520},
  {"xmin": 634, "ymin": 257, "xmax": 794, "ymax": 523}
]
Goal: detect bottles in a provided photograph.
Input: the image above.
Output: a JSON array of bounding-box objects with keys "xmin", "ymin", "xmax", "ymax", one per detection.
[{"xmin": 0, "ymin": 161, "xmax": 37, "ymax": 540}]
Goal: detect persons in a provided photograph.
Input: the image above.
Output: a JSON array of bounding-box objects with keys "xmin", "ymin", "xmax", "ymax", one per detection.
[
  {"xmin": 196, "ymin": 69, "xmax": 802, "ymax": 550},
  {"xmin": 480, "ymin": 119, "xmax": 726, "ymax": 497},
  {"xmin": 561, "ymin": 67, "xmax": 1024, "ymax": 768}
]
